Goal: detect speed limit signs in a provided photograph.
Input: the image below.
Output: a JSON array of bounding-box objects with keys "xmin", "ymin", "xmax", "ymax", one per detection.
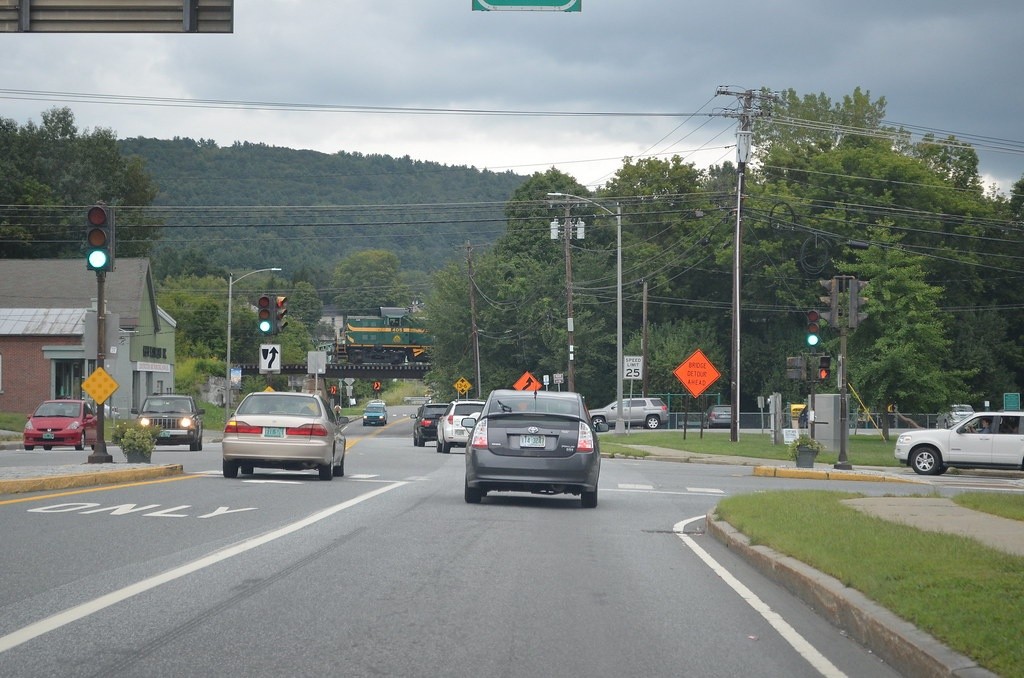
[{"xmin": 623, "ymin": 355, "xmax": 644, "ymax": 380}]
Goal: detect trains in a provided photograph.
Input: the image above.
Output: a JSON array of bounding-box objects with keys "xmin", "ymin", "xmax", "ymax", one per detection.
[{"xmin": 327, "ymin": 306, "xmax": 434, "ymax": 364}]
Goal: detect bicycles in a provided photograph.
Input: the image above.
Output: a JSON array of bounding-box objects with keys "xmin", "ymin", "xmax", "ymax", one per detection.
[{"xmin": 335, "ymin": 411, "xmax": 340, "ymax": 420}]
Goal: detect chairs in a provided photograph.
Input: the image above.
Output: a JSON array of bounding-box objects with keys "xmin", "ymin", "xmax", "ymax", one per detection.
[
  {"xmin": 47, "ymin": 406, "xmax": 79, "ymax": 416},
  {"xmin": 148, "ymin": 400, "xmax": 188, "ymax": 411},
  {"xmin": 257, "ymin": 401, "xmax": 314, "ymax": 414}
]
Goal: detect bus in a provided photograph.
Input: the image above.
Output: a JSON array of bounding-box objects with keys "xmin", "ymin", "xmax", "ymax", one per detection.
[{"xmin": 403, "ymin": 397, "xmax": 431, "ymax": 404}]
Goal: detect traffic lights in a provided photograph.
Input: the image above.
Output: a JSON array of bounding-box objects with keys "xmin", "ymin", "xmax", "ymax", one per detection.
[
  {"xmin": 849, "ymin": 280, "xmax": 870, "ymax": 327},
  {"xmin": 787, "ymin": 356, "xmax": 805, "ymax": 381},
  {"xmin": 807, "ymin": 310, "xmax": 822, "ymax": 347},
  {"xmin": 256, "ymin": 296, "xmax": 273, "ymax": 335},
  {"xmin": 86, "ymin": 207, "xmax": 112, "ymax": 271},
  {"xmin": 817, "ymin": 356, "xmax": 830, "ymax": 381},
  {"xmin": 274, "ymin": 296, "xmax": 289, "ymax": 334},
  {"xmin": 819, "ymin": 279, "xmax": 838, "ymax": 327}
]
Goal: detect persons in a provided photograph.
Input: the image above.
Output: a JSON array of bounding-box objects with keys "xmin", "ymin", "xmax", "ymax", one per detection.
[
  {"xmin": 970, "ymin": 418, "xmax": 991, "ymax": 434},
  {"xmin": 335, "ymin": 403, "xmax": 342, "ymax": 417}
]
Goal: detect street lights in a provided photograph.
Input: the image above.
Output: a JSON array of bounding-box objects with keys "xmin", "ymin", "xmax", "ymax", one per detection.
[
  {"xmin": 547, "ymin": 192, "xmax": 625, "ymax": 433},
  {"xmin": 225, "ymin": 267, "xmax": 282, "ymax": 421}
]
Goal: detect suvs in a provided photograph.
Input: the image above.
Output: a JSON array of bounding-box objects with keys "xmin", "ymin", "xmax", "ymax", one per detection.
[
  {"xmin": 131, "ymin": 393, "xmax": 205, "ymax": 451},
  {"xmin": 703, "ymin": 404, "xmax": 731, "ymax": 428},
  {"xmin": 409, "ymin": 400, "xmax": 449, "ymax": 447},
  {"xmin": 435, "ymin": 400, "xmax": 486, "ymax": 453},
  {"xmin": 936, "ymin": 405, "xmax": 975, "ymax": 429},
  {"xmin": 361, "ymin": 400, "xmax": 388, "ymax": 426},
  {"xmin": 588, "ymin": 398, "xmax": 670, "ymax": 429},
  {"xmin": 893, "ymin": 410, "xmax": 1024, "ymax": 475}
]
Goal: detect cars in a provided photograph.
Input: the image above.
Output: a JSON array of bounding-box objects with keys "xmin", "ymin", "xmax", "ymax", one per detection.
[
  {"xmin": 222, "ymin": 392, "xmax": 349, "ymax": 481},
  {"xmin": 461, "ymin": 388, "xmax": 609, "ymax": 508},
  {"xmin": 23, "ymin": 400, "xmax": 98, "ymax": 450}
]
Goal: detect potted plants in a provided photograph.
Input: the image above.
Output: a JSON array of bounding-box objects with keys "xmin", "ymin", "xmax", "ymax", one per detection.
[{"xmin": 788, "ymin": 434, "xmax": 826, "ymax": 469}]
